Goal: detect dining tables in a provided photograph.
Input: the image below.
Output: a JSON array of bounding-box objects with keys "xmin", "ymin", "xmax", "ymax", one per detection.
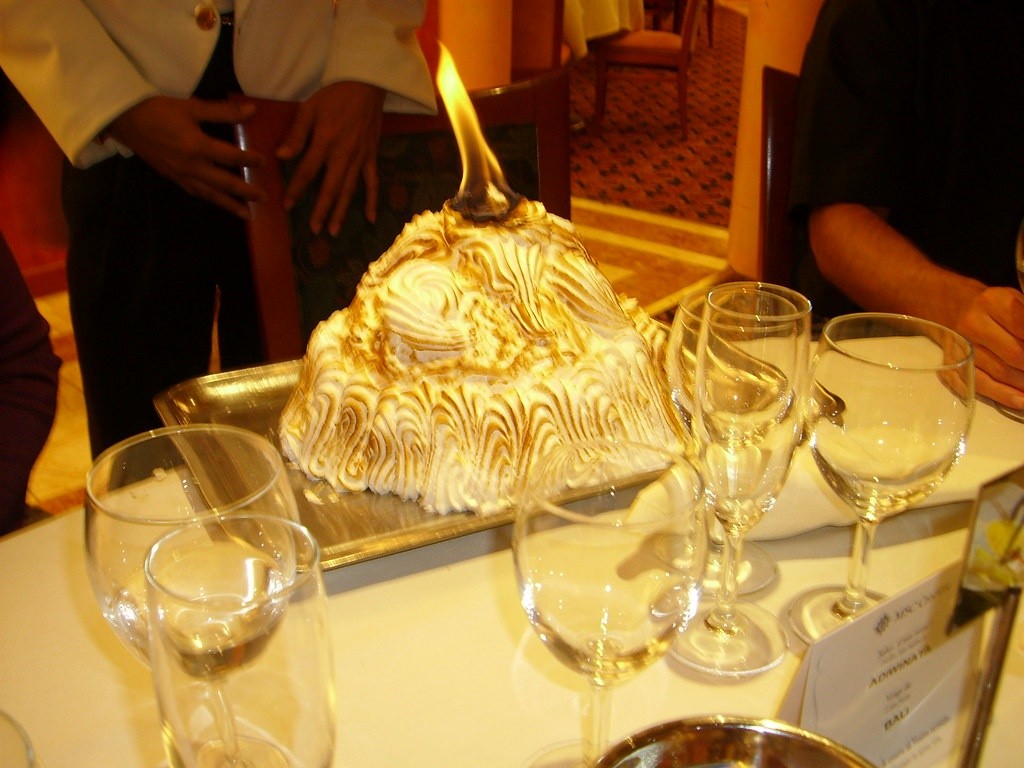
[{"xmin": 0, "ymin": 332, "xmax": 1024, "ymax": 768}]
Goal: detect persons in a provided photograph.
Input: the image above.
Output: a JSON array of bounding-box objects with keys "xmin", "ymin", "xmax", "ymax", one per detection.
[
  {"xmin": 770, "ymin": 0, "xmax": 1024, "ymax": 411},
  {"xmin": 0, "ymin": 0, "xmax": 440, "ymax": 465},
  {"xmin": 1, "ymin": 230, "xmax": 62, "ymax": 540}
]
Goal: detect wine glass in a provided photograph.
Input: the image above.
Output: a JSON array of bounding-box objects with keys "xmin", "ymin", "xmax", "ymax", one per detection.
[
  {"xmin": 85, "ymin": 424, "xmax": 306, "ymax": 768},
  {"xmin": 668, "ymin": 290, "xmax": 814, "ymax": 591},
  {"xmin": 787, "ymin": 311, "xmax": 976, "ymax": 652},
  {"xmin": 673, "ymin": 288, "xmax": 811, "ymax": 677},
  {"xmin": 512, "ymin": 439, "xmax": 709, "ymax": 768}
]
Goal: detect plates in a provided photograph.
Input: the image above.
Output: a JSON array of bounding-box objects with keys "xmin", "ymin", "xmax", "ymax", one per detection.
[{"xmin": 594, "ymin": 716, "xmax": 880, "ymax": 767}]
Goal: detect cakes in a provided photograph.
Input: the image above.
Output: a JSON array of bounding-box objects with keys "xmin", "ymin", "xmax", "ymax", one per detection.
[{"xmin": 273, "ymin": 196, "xmax": 706, "ymax": 514}]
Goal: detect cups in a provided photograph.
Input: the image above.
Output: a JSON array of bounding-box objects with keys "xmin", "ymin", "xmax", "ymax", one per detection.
[{"xmin": 143, "ymin": 512, "xmax": 337, "ymax": 768}]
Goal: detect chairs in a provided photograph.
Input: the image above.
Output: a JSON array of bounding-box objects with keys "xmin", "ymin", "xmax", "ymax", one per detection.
[
  {"xmin": 581, "ymin": 0, "xmax": 700, "ymax": 140},
  {"xmin": 230, "ymin": 71, "xmax": 575, "ymax": 368}
]
